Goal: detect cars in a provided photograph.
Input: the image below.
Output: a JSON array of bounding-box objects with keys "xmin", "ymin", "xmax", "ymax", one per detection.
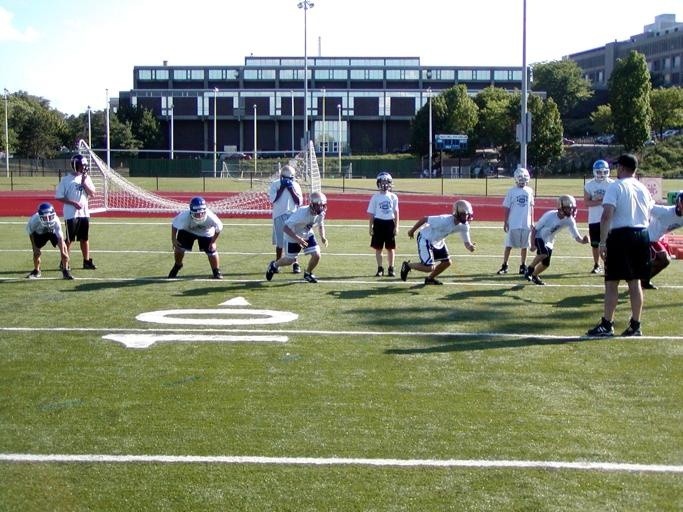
[
  {"xmin": 597, "ymin": 129, "xmax": 680, "ymax": 145},
  {"xmin": 257, "ymin": 148, "xmax": 286, "ymax": 158},
  {"xmin": 562, "ymin": 137, "xmax": 575, "ymax": 145},
  {"xmin": 163, "ymin": 147, "xmax": 200, "ymax": 159},
  {"xmin": 224, "ymin": 152, "xmax": 251, "ymax": 160},
  {"xmin": 391, "ymin": 143, "xmax": 415, "ymax": 154}
]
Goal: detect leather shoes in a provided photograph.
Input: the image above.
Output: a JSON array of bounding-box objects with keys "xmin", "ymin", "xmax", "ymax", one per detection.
[{"xmin": 168, "ymin": 262, "xmax": 183, "ymax": 278}]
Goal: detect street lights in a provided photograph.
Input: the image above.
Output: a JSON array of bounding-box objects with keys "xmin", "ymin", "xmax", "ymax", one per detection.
[
  {"xmin": 86, "ymin": 104, "xmax": 92, "ymax": 176},
  {"xmin": 169, "ymin": 103, "xmax": 175, "ymax": 159},
  {"xmin": 296, "ymin": 0, "xmax": 316, "ymax": 149},
  {"xmin": 320, "ymin": 86, "xmax": 328, "ymax": 178},
  {"xmin": 252, "ymin": 103, "xmax": 257, "ymax": 175},
  {"xmin": 0, "ymin": 88, "xmax": 10, "ymax": 179},
  {"xmin": 336, "ymin": 103, "xmax": 342, "ymax": 174},
  {"xmin": 211, "ymin": 86, "xmax": 220, "ymax": 177},
  {"xmin": 425, "ymin": 85, "xmax": 432, "ymax": 178},
  {"xmin": 289, "ymin": 88, "xmax": 296, "ymax": 157},
  {"xmin": 104, "ymin": 88, "xmax": 113, "ymax": 169}
]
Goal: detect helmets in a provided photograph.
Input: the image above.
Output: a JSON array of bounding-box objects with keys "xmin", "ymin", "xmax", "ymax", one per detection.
[
  {"xmin": 514, "ymin": 167, "xmax": 530, "ymax": 187},
  {"xmin": 376, "ymin": 171, "xmax": 393, "ymax": 192},
  {"xmin": 556, "ymin": 194, "xmax": 577, "ymax": 218},
  {"xmin": 675, "ymin": 191, "xmax": 683, "ymax": 216},
  {"xmin": 189, "ymin": 196, "xmax": 208, "ymax": 225},
  {"xmin": 70, "ymin": 154, "xmax": 90, "ymax": 176},
  {"xmin": 280, "ymin": 165, "xmax": 296, "ymax": 185},
  {"xmin": 309, "ymin": 192, "xmax": 328, "ymax": 216},
  {"xmin": 593, "ymin": 160, "xmax": 610, "ymax": 182},
  {"xmin": 38, "ymin": 203, "xmax": 57, "ymax": 228},
  {"xmin": 452, "ymin": 200, "xmax": 474, "ymax": 225}
]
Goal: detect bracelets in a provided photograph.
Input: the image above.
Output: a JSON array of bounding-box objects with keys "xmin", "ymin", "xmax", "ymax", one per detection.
[{"xmin": 599, "ymin": 241, "xmax": 607, "ymax": 247}]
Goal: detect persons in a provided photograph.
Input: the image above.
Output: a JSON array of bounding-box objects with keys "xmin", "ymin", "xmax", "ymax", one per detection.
[
  {"xmin": 266, "ymin": 191, "xmax": 329, "ymax": 282},
  {"xmin": 268, "ymin": 166, "xmax": 304, "ymax": 274},
  {"xmin": 583, "ymin": 160, "xmax": 615, "ymax": 274},
  {"xmin": 168, "ymin": 197, "xmax": 224, "ymax": 279},
  {"xmin": 400, "ymin": 200, "xmax": 476, "ymax": 285},
  {"xmin": 585, "ymin": 154, "xmax": 653, "ymax": 335},
  {"xmin": 641, "ymin": 191, "xmax": 683, "ymax": 290},
  {"xmin": 496, "ymin": 168, "xmax": 535, "ymax": 274},
  {"xmin": 367, "ymin": 172, "xmax": 399, "ymax": 276},
  {"xmin": 55, "ymin": 154, "xmax": 97, "ymax": 270},
  {"xmin": 524, "ymin": 194, "xmax": 589, "ymax": 285},
  {"xmin": 26, "ymin": 202, "xmax": 76, "ymax": 279}
]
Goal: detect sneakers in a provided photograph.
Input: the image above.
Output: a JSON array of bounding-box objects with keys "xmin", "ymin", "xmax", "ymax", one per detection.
[
  {"xmin": 588, "ymin": 317, "xmax": 615, "ymax": 336},
  {"xmin": 592, "ymin": 263, "xmax": 602, "ymax": 273},
  {"xmin": 497, "ymin": 262, "xmax": 509, "ymax": 275},
  {"xmin": 376, "ymin": 266, "xmax": 384, "ymax": 276},
  {"xmin": 520, "ymin": 262, "xmax": 544, "ymax": 285},
  {"xmin": 59, "ymin": 258, "xmax": 97, "ymax": 279},
  {"xmin": 641, "ymin": 280, "xmax": 658, "ymax": 289},
  {"xmin": 266, "ymin": 260, "xmax": 318, "ymax": 283},
  {"xmin": 27, "ymin": 269, "xmax": 42, "ymax": 279},
  {"xmin": 212, "ymin": 268, "xmax": 224, "ymax": 279},
  {"xmin": 388, "ymin": 266, "xmax": 395, "ymax": 276},
  {"xmin": 425, "ymin": 276, "xmax": 443, "ymax": 285},
  {"xmin": 621, "ymin": 317, "xmax": 642, "ymax": 336},
  {"xmin": 401, "ymin": 260, "xmax": 411, "ymax": 281}
]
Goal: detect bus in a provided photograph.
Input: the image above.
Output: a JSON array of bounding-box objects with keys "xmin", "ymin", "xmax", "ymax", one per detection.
[{"xmin": 474, "ymin": 149, "xmax": 500, "ymax": 165}]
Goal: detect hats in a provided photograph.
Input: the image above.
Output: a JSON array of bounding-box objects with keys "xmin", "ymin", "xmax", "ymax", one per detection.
[{"xmin": 612, "ymin": 155, "xmax": 638, "ymax": 171}]
